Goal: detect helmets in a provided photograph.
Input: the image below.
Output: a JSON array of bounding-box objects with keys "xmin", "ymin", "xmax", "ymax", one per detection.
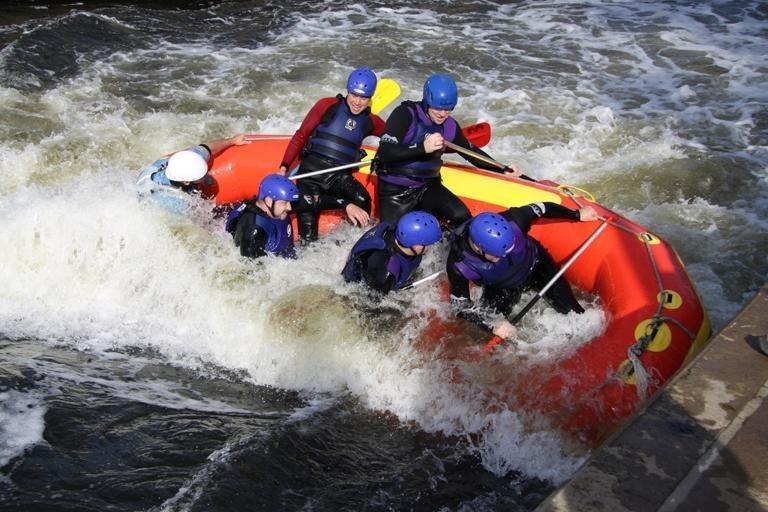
[
  {"xmin": 395, "ymin": 211, "xmax": 441, "ymax": 247},
  {"xmin": 469, "ymin": 212, "xmax": 516, "ymax": 257},
  {"xmin": 422, "ymin": 75, "xmax": 457, "ymax": 113},
  {"xmin": 258, "ymin": 174, "xmax": 300, "ymax": 201},
  {"xmin": 165, "ymin": 150, "xmax": 208, "ymax": 181},
  {"xmin": 347, "ymin": 68, "xmax": 377, "ymax": 96}
]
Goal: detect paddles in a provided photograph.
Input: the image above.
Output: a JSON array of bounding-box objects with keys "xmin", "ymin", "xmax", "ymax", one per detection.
[
  {"xmin": 288, "ymin": 122, "xmax": 493, "ymax": 179},
  {"xmin": 288, "ymin": 78, "xmax": 401, "ymax": 175}
]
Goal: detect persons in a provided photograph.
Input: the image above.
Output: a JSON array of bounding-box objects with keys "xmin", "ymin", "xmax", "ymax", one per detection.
[
  {"xmin": 233, "ymin": 172, "xmax": 372, "ymax": 261},
  {"xmin": 277, "ymin": 69, "xmax": 385, "ymax": 246},
  {"xmin": 445, "ymin": 201, "xmax": 598, "ymax": 344},
  {"xmin": 342, "ymin": 211, "xmax": 443, "ymax": 311},
  {"xmin": 374, "ymin": 73, "xmax": 524, "ymax": 233},
  {"xmin": 136, "ymin": 133, "xmax": 253, "ymax": 219}
]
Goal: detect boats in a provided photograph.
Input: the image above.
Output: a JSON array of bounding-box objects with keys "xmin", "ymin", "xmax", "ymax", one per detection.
[{"xmin": 130, "ymin": 132, "xmax": 713, "ymax": 488}]
